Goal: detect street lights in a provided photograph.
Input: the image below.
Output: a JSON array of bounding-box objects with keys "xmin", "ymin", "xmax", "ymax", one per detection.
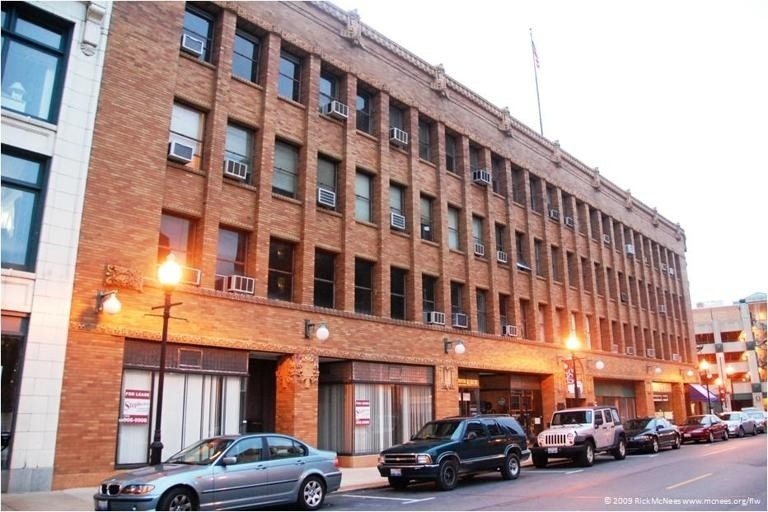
[
  {"xmin": 137, "ymin": 251, "xmax": 189, "ymax": 470},
  {"xmin": 726, "ymin": 365, "xmax": 735, "ymax": 411},
  {"xmin": 698, "ymin": 359, "xmax": 713, "ymax": 415},
  {"xmin": 562, "ymin": 332, "xmax": 586, "ymax": 409}
]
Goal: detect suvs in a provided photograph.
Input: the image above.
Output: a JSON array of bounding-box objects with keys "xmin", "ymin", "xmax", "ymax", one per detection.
[
  {"xmin": 377, "ymin": 411, "xmax": 532, "ymax": 493},
  {"xmin": 526, "ymin": 403, "xmax": 626, "ymax": 470}
]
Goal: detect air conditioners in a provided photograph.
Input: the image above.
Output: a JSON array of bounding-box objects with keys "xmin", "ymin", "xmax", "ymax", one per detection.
[
  {"xmin": 317, "ymin": 188, "xmax": 335, "ymax": 207},
  {"xmin": 179, "ymin": 267, "xmax": 254, "ymax": 294},
  {"xmin": 324, "ymin": 100, "xmax": 349, "ymax": 120},
  {"xmin": 603, "ymin": 234, "xmax": 636, "ymax": 256},
  {"xmin": 391, "ymin": 213, "xmax": 405, "ymax": 230},
  {"xmin": 472, "ymin": 170, "xmax": 491, "ymax": 185},
  {"xmin": 659, "ymin": 305, "xmax": 666, "ymax": 313},
  {"xmin": 389, "ymin": 127, "xmax": 408, "ymax": 146},
  {"xmin": 662, "ymin": 263, "xmax": 674, "ymax": 276},
  {"xmin": 167, "ymin": 141, "xmax": 194, "ymax": 164},
  {"xmin": 181, "ymin": 34, "xmax": 204, "ymax": 56},
  {"xmin": 611, "ymin": 344, "xmax": 682, "ymax": 362},
  {"xmin": 503, "ymin": 323, "xmax": 518, "ymax": 337},
  {"xmin": 425, "ymin": 311, "xmax": 469, "ymax": 328},
  {"xmin": 550, "ymin": 209, "xmax": 573, "ymax": 227},
  {"xmin": 474, "ymin": 243, "xmax": 507, "ymax": 263},
  {"xmin": 223, "ymin": 159, "xmax": 247, "ymax": 181}
]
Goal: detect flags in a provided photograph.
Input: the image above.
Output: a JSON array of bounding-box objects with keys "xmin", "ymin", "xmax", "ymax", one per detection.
[{"xmin": 532, "ymin": 44, "xmax": 541, "ymax": 70}]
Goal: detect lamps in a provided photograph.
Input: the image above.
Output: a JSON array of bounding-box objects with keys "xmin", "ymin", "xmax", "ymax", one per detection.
[
  {"xmin": 97, "ymin": 288, "xmax": 122, "ymax": 316},
  {"xmin": 646, "ymin": 364, "xmax": 661, "ymax": 374},
  {"xmin": 444, "ymin": 338, "xmax": 465, "ymax": 354},
  {"xmin": 587, "ymin": 359, "xmax": 604, "ymax": 369},
  {"xmin": 305, "ymin": 320, "xmax": 329, "ymax": 340}
]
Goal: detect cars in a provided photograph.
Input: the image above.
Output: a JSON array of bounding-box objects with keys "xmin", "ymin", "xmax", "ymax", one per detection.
[
  {"xmin": 741, "ymin": 407, "xmax": 767, "ymax": 433},
  {"xmin": 718, "ymin": 411, "xmax": 757, "ymax": 438},
  {"xmin": 621, "ymin": 416, "xmax": 682, "ymax": 453},
  {"xmin": 94, "ymin": 430, "xmax": 344, "ymax": 511},
  {"xmin": 676, "ymin": 413, "xmax": 728, "ymax": 444}
]
